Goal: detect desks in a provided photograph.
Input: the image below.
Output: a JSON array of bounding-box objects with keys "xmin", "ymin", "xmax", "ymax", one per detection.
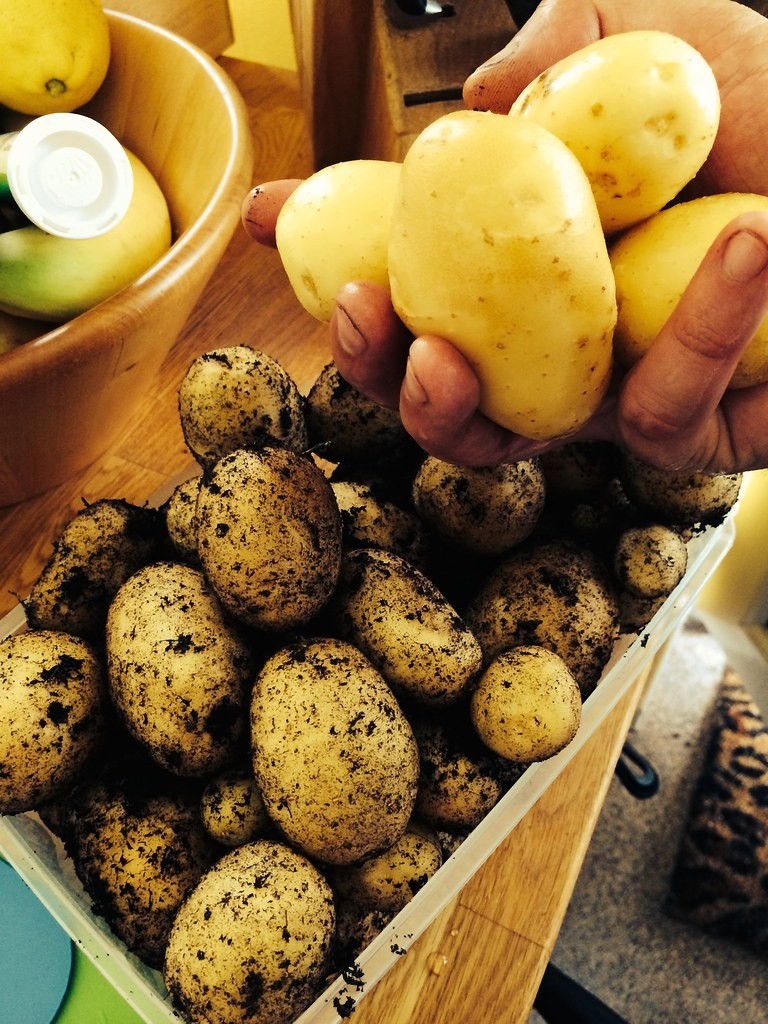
[{"xmin": 2, "ymin": 54, "xmax": 666, "ymax": 1024}]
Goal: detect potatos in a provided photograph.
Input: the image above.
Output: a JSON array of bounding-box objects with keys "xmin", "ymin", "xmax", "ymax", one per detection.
[{"xmin": 1, "ymin": 33, "xmax": 768, "ymax": 1024}]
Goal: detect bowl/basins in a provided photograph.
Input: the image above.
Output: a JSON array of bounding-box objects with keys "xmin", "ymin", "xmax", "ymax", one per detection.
[{"xmin": 0, "ymin": 8, "xmax": 255, "ymax": 509}]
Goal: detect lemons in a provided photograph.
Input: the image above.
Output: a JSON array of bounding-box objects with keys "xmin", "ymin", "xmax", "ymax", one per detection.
[{"xmin": 1, "ymin": 0, "xmax": 112, "ymax": 117}]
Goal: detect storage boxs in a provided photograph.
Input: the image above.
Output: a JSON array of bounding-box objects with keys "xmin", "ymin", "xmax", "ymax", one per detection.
[{"xmin": 1, "ymin": 518, "xmax": 737, "ymax": 1024}]
